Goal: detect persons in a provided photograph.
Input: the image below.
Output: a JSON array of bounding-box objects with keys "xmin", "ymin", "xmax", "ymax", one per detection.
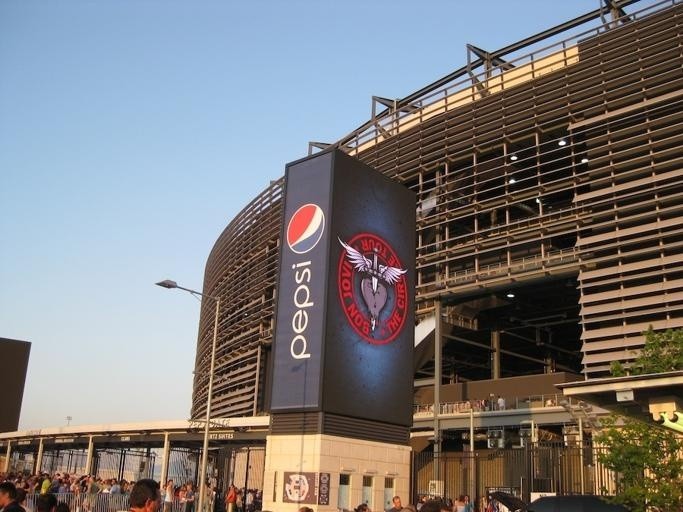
[
  {"xmin": 521, "ymin": 398, "xmax": 556, "ymax": 407},
  {"xmin": 0, "ymin": 469, "xmax": 262, "ymax": 512},
  {"xmin": 466, "ymin": 395, "xmax": 507, "ymax": 414},
  {"xmin": 297, "ymin": 507, "xmax": 313, "ymax": 512},
  {"xmin": 336, "ymin": 492, "xmax": 500, "ymax": 512}
]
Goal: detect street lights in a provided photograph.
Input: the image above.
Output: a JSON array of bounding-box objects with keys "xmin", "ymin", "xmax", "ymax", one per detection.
[{"xmin": 156, "ymin": 279, "xmax": 220, "ymax": 509}]
